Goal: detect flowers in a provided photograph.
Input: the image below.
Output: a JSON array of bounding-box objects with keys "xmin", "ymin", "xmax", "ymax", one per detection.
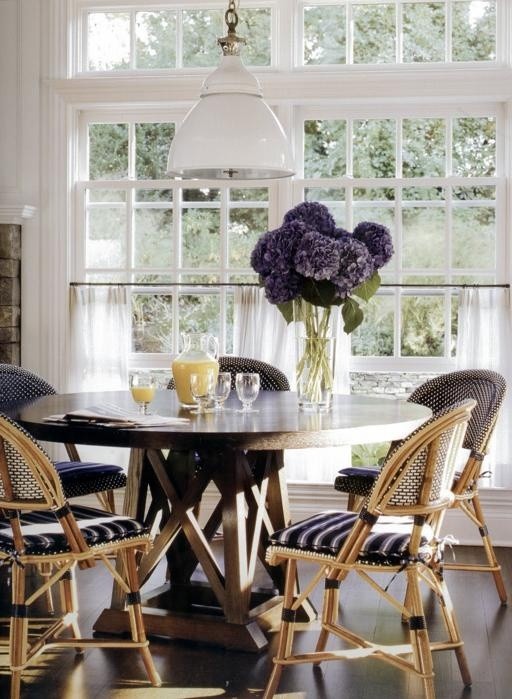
[{"xmin": 249, "ymin": 201, "xmax": 396, "ymax": 405}]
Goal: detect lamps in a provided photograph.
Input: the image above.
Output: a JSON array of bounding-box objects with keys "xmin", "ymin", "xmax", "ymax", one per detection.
[{"xmin": 165, "ymin": 0, "xmax": 296, "ymax": 179}]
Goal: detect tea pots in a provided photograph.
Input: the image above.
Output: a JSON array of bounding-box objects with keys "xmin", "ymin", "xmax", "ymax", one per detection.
[{"xmin": 171, "ymin": 331, "xmax": 221, "ymax": 407}]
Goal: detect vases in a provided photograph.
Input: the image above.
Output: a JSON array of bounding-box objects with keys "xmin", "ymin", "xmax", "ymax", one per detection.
[{"xmin": 294, "ymin": 333, "xmax": 337, "ymax": 414}]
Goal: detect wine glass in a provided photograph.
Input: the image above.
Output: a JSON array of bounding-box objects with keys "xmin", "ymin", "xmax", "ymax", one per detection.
[
  {"xmin": 131, "ymin": 372, "xmax": 155, "ymax": 415},
  {"xmin": 189, "ymin": 373, "xmax": 213, "ymax": 416},
  {"xmin": 234, "ymin": 373, "xmax": 262, "ymax": 414},
  {"xmin": 209, "ymin": 372, "xmax": 235, "ymax": 413}
]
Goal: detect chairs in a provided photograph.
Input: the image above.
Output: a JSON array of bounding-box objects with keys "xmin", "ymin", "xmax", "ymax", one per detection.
[
  {"xmin": 0, "ymin": 412, "xmax": 163, "ymax": 699},
  {"xmin": 166, "ymin": 356, "xmax": 289, "ymax": 520},
  {"xmin": 261, "ymin": 396, "xmax": 478, "ymax": 699},
  {"xmin": 325, "ymin": 368, "xmax": 509, "ymax": 606},
  {"xmin": 0, "ymin": 364, "xmax": 126, "ymax": 616}
]
